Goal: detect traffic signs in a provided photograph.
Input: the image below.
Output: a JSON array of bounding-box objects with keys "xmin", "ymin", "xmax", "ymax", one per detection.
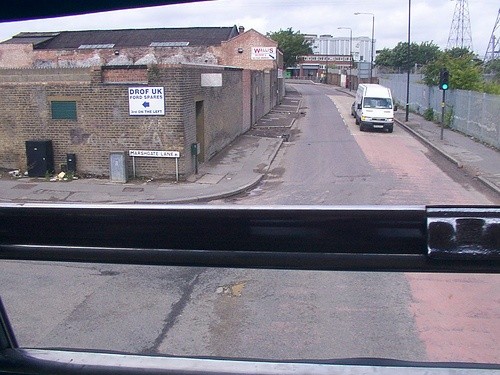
[{"xmin": 128, "ymin": 87, "xmax": 165, "ymax": 117}]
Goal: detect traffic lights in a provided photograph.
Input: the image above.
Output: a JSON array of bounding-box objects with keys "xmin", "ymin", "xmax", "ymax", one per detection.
[{"xmin": 439, "ymin": 68, "xmax": 449, "ymax": 90}]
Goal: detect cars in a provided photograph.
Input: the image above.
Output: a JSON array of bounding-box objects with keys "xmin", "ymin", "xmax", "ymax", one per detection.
[{"xmin": 351, "ymin": 101, "xmax": 356, "ymax": 119}]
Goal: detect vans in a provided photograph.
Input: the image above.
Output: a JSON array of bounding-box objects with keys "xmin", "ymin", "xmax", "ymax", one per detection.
[{"xmin": 354, "ymin": 84, "xmax": 395, "ymax": 133}]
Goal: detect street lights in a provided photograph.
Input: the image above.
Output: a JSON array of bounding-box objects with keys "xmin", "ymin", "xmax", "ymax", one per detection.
[
  {"xmin": 338, "ymin": 27, "xmax": 352, "ymax": 92},
  {"xmin": 354, "ymin": 12, "xmax": 375, "ymax": 84}
]
